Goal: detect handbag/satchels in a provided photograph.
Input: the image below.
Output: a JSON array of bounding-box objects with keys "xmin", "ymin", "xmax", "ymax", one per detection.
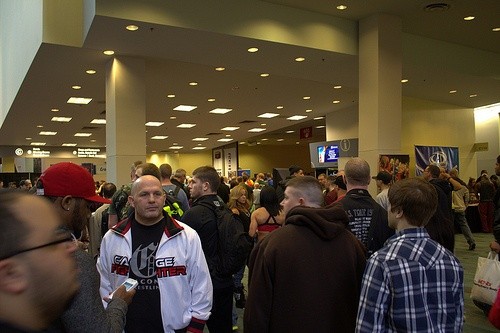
[{"xmin": 470, "ymin": 251, "xmax": 500, "ymax": 302}]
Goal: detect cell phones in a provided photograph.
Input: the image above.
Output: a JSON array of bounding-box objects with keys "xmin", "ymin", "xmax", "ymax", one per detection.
[{"xmin": 108, "ymin": 278, "xmax": 138, "ymax": 300}]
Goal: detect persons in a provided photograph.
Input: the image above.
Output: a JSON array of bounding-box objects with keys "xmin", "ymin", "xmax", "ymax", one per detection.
[
  {"xmin": 468, "ymin": 170, "xmax": 498, "ymax": 233},
  {"xmin": 96, "ymin": 175, "xmax": 214, "ymax": 333},
  {"xmin": 27, "ymin": 163, "xmax": 135, "ymax": 333},
  {"xmin": 355, "ymin": 178, "xmax": 464, "ymax": 333},
  {"xmin": 182, "ymin": 166, "xmax": 232, "ymax": 333},
  {"xmin": 243, "ymin": 177, "xmax": 366, "ymax": 332},
  {"xmin": 0, "ymin": 156, "xmax": 476, "ymax": 309},
  {"xmin": 473, "ymin": 155, "xmax": 500, "ymax": 316},
  {"xmin": 0, "ymin": 189, "xmax": 79, "ymax": 332}
]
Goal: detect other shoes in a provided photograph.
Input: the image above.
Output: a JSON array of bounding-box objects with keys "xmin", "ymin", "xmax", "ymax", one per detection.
[
  {"xmin": 468, "ymin": 243, "xmax": 476, "ymax": 251},
  {"xmin": 233, "ymin": 328, "xmax": 239, "ymax": 333}
]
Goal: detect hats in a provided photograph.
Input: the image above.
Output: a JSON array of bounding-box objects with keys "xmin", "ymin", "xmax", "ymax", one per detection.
[
  {"xmin": 36, "ymin": 162, "xmax": 113, "ymax": 204},
  {"xmin": 372, "ymin": 170, "xmax": 393, "ymax": 183},
  {"xmin": 289, "ymin": 166, "xmax": 302, "ymax": 176}
]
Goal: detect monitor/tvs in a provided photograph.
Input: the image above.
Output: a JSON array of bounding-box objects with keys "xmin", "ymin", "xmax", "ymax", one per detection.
[{"xmin": 309, "ymin": 139, "xmax": 341, "ymax": 169}]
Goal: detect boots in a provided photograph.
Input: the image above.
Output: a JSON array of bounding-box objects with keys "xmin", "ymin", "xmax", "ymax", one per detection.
[{"xmin": 232, "ymin": 283, "xmax": 247, "ymax": 309}]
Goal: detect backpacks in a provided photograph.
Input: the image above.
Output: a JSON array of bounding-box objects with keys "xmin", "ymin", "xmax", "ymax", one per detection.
[
  {"xmin": 192, "ymin": 195, "xmax": 255, "ymax": 289},
  {"xmin": 162, "ymin": 186, "xmax": 188, "ymax": 220}
]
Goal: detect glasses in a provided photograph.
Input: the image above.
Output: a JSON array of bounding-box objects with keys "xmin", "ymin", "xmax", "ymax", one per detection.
[{"xmin": 0, "ymin": 226, "xmax": 75, "ymax": 260}]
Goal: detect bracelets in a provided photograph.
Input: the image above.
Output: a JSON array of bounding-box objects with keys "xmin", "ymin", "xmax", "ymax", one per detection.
[{"xmin": 447, "ymin": 177, "xmax": 451, "ymax": 181}]
